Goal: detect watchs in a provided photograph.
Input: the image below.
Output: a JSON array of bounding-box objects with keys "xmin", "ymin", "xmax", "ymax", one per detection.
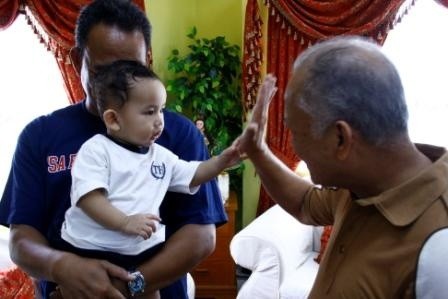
[{"xmin": 127, "ymin": 271, "xmax": 145, "ymax": 297}]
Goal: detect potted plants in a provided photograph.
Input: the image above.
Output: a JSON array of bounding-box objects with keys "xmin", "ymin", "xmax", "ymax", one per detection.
[{"xmin": 165, "ymin": 27, "xmax": 242, "ymax": 204}]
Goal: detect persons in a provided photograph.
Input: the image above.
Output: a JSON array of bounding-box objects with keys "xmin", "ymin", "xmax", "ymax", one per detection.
[
  {"xmin": 60, "ymin": 60, "xmax": 250, "ymax": 299},
  {"xmin": 239, "ymin": 34, "xmax": 448, "ymax": 299},
  {"xmin": 0, "ymin": 0, "xmax": 228, "ymax": 299}
]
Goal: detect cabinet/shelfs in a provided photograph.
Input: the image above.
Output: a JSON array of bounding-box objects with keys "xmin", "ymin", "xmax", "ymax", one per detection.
[{"xmin": 189, "ymin": 188, "xmax": 238, "ymax": 299}]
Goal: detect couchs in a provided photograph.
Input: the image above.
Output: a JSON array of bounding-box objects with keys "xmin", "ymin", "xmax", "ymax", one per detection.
[{"xmin": 229, "ymin": 203, "xmax": 322, "ymax": 299}]
